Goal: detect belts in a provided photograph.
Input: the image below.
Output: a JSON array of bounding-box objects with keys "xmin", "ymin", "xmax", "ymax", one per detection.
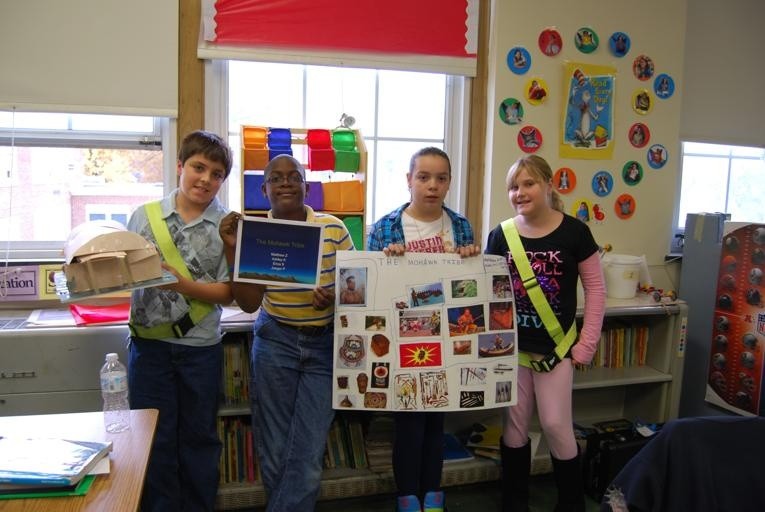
[{"xmin": 296, "ymin": 325, "xmax": 332, "ymax": 338}]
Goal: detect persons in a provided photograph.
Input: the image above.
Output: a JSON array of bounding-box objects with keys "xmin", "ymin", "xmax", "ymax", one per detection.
[
  {"xmin": 340, "ymin": 273, "xmax": 363, "ymax": 303},
  {"xmin": 483, "ymin": 155, "xmax": 607, "ymax": 511},
  {"xmin": 60, "ymin": 128, "xmax": 236, "ymax": 512},
  {"xmin": 365, "ymin": 146, "xmax": 482, "ymax": 511},
  {"xmin": 457, "ymin": 306, "xmax": 478, "ymax": 334},
  {"xmin": 217, "ymin": 155, "xmax": 360, "ymax": 512}
]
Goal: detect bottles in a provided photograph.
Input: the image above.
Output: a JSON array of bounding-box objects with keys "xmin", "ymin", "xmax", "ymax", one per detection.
[
  {"xmin": 99, "ymin": 352, "xmax": 132, "ymax": 434},
  {"xmin": 54, "ymin": 271, "xmax": 71, "ymax": 303}
]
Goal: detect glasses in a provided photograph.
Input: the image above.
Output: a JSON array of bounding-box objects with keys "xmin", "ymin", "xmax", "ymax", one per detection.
[{"xmin": 263, "ymin": 173, "xmax": 305, "ymax": 186}]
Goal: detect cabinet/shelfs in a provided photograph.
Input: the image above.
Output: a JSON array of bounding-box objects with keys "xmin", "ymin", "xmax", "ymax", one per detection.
[{"xmin": 216, "ymin": 292, "xmax": 690, "ymax": 511}]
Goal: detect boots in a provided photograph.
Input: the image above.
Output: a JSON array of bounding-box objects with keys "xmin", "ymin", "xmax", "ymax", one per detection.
[
  {"xmin": 395, "ymin": 489, "xmax": 446, "ymax": 511},
  {"xmin": 550, "ymin": 442, "xmax": 587, "ymax": 511},
  {"xmin": 499, "ymin": 435, "xmax": 532, "ymax": 511}
]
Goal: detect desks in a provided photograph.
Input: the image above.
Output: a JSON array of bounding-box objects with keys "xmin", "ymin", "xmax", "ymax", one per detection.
[{"xmin": 0, "ymin": 409, "xmax": 161, "ymax": 512}]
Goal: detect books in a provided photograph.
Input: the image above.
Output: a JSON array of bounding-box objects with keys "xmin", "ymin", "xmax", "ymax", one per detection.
[
  {"xmin": 575, "ymin": 328, "xmax": 649, "ymax": 370},
  {"xmin": 438, "ymin": 409, "xmax": 508, "ymax": 463},
  {"xmin": 321, "ymin": 411, "xmax": 396, "ymax": 470},
  {"xmin": 221, "ymin": 331, "xmax": 255, "ymax": 408},
  {"xmin": 220, "ymin": 416, "xmax": 262, "ymax": 484},
  {"xmin": 0, "ymin": 439, "xmax": 113, "ymax": 501}
]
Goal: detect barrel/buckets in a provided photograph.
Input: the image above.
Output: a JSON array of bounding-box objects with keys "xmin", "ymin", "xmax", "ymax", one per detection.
[{"xmin": 600, "ymin": 253, "xmax": 643, "ymax": 300}]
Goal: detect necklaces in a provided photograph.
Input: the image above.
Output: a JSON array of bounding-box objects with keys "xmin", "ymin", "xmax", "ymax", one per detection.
[{"xmin": 407, "ymin": 203, "xmax": 445, "ymax": 254}]
